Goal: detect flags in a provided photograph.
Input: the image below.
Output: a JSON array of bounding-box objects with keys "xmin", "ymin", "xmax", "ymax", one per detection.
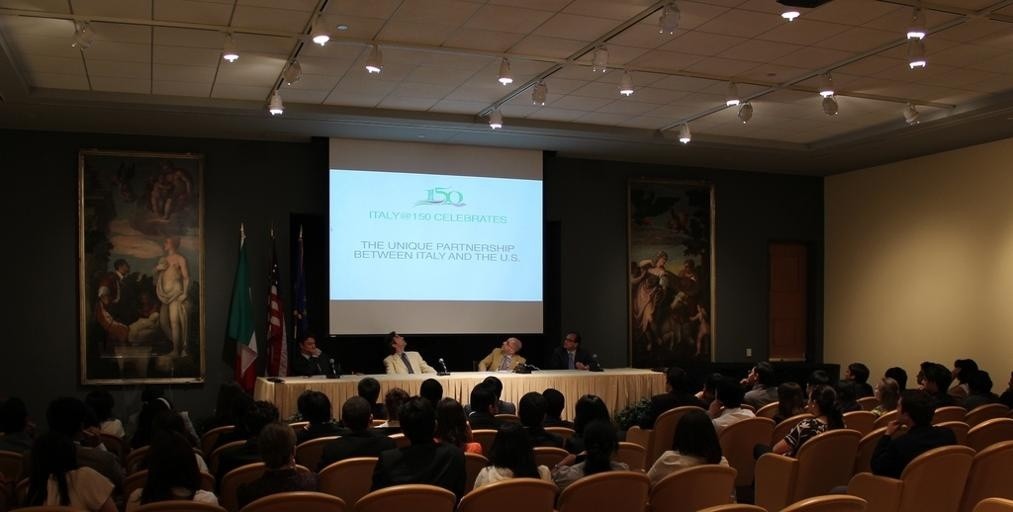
[
  {"xmin": 291, "ymin": 237, "xmax": 309, "ymax": 359},
  {"xmin": 225, "ymin": 234, "xmax": 259, "ymax": 393},
  {"xmin": 264, "ymin": 233, "xmax": 290, "ymax": 378}
]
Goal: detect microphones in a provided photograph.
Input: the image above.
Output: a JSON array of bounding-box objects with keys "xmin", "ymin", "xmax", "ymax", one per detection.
[
  {"xmin": 438, "ymin": 358, "xmax": 450, "ymax": 375},
  {"xmin": 512, "ymin": 362, "xmax": 531, "ymax": 373},
  {"xmin": 592, "ymin": 354, "xmax": 601, "ymax": 371},
  {"xmin": 330, "ymin": 359, "xmax": 337, "ymax": 377}
]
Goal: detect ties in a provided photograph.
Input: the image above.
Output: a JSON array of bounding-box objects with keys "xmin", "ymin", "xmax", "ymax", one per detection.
[
  {"xmin": 400, "ymin": 353, "xmax": 414, "ymax": 375},
  {"xmin": 568, "ymin": 352, "xmax": 574, "ymax": 368},
  {"xmin": 502, "ymin": 356, "xmax": 508, "ymax": 369}
]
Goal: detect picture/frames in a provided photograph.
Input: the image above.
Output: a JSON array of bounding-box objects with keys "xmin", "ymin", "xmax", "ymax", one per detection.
[
  {"xmin": 627, "ymin": 179, "xmax": 715, "ymax": 367},
  {"xmin": 78, "ymin": 149, "xmax": 206, "ymax": 384}
]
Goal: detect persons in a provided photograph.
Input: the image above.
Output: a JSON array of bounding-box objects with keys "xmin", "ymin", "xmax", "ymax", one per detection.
[
  {"xmin": 477, "ymin": 337, "xmax": 526, "ymax": 371},
  {"xmin": 289, "ymin": 336, "xmax": 341, "ymax": 376},
  {"xmin": 383, "ymin": 331, "xmax": 437, "ymax": 374},
  {"xmin": 630, "ymin": 250, "xmax": 710, "ymax": 358},
  {"xmin": 552, "ymin": 331, "xmax": 600, "ymax": 370},
  {"xmin": 0, "ymin": 360, "xmax": 1013, "ymax": 511},
  {"xmin": 91, "ymin": 160, "xmax": 193, "ymax": 366}
]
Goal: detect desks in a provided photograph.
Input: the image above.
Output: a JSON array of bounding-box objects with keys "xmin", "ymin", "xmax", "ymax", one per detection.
[{"xmin": 253, "ymin": 367, "xmax": 667, "ymax": 425}]
